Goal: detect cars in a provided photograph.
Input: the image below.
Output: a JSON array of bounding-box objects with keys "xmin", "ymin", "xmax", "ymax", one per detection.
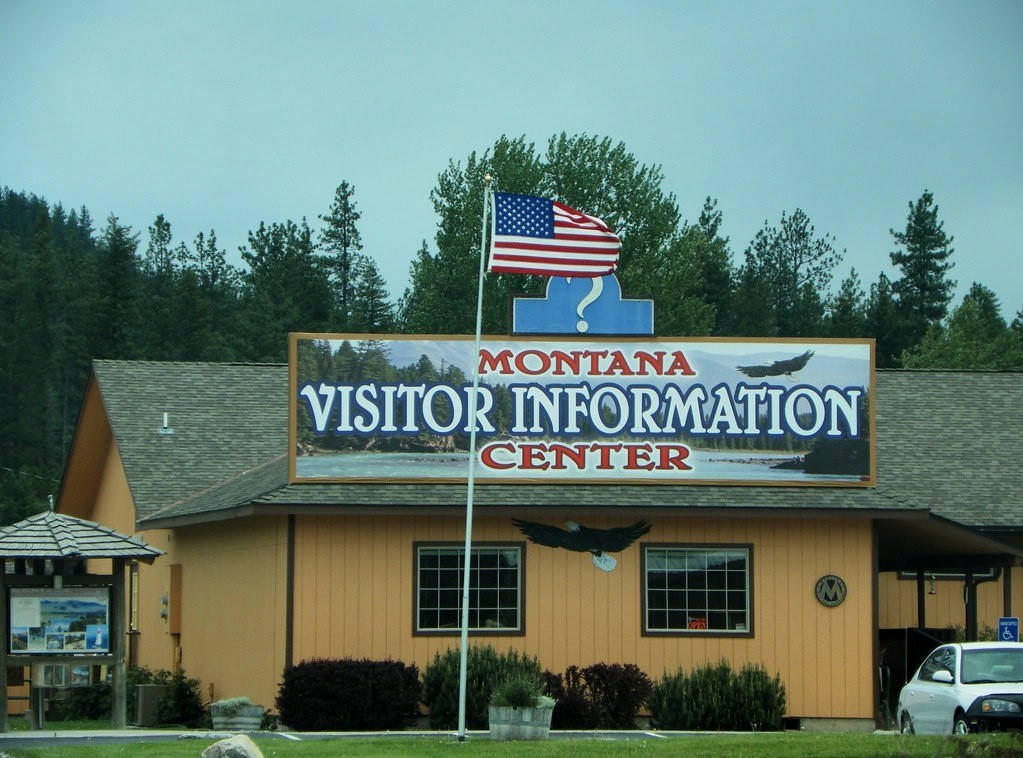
[{"xmin": 896, "ymin": 641, "xmax": 1023, "ymax": 734}]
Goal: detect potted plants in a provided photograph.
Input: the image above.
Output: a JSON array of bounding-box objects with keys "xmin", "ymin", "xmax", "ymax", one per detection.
[
  {"xmin": 209, "ymin": 698, "xmax": 263, "ymax": 730},
  {"xmin": 488, "ymin": 672, "xmax": 557, "ymax": 741}
]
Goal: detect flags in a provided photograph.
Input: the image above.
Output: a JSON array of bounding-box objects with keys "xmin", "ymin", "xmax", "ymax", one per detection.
[{"xmin": 488, "ymin": 191, "xmax": 622, "ymax": 277}]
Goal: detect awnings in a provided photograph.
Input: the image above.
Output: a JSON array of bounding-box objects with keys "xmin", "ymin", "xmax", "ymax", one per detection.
[{"xmin": 880, "ymin": 513, "xmax": 1023, "ymax": 568}]
